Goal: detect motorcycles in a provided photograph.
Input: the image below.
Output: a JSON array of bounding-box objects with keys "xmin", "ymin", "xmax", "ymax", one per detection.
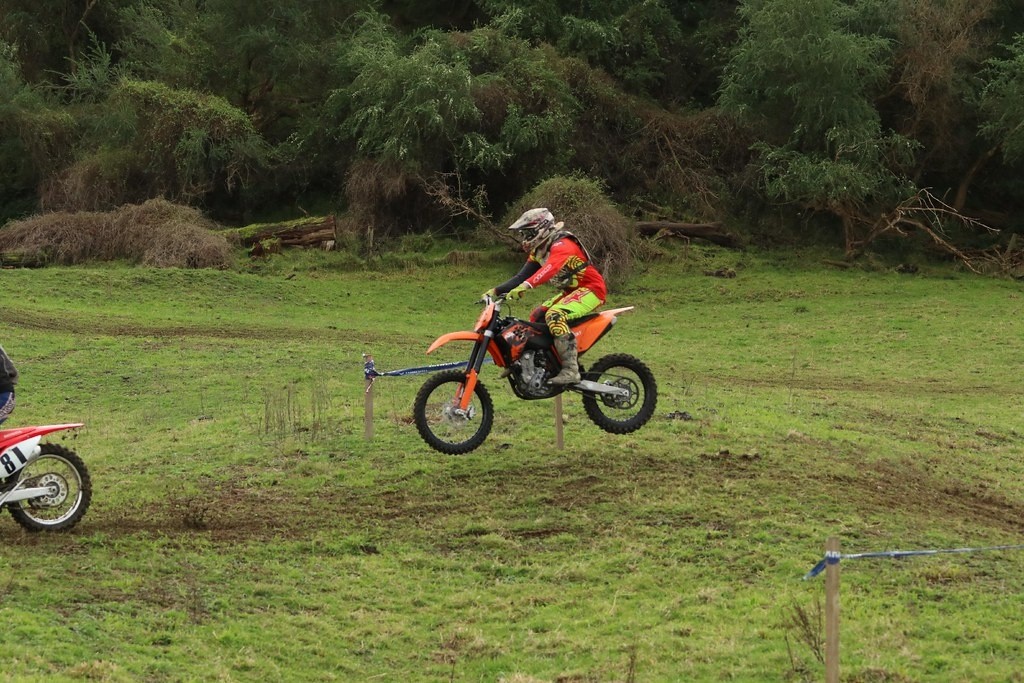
[
  {"xmin": 412, "ymin": 294, "xmax": 658, "ymax": 457},
  {"xmin": 0, "ymin": 417, "xmax": 91, "ymax": 530}
]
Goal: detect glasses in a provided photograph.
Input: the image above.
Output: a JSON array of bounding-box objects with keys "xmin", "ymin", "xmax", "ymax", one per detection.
[{"xmin": 519, "ymin": 213, "xmax": 554, "ymax": 241}]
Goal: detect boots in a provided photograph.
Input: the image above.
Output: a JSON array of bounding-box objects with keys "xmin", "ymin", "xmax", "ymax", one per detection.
[
  {"xmin": 547, "ymin": 331, "xmax": 581, "ymax": 385},
  {"xmin": 501, "ymin": 368, "xmax": 511, "ymax": 378}
]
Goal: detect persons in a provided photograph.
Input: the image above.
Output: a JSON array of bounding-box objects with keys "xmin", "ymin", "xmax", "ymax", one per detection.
[
  {"xmin": 0, "ymin": 346, "xmax": 19, "ymax": 424},
  {"xmin": 481, "ymin": 208, "xmax": 607, "ymax": 384}
]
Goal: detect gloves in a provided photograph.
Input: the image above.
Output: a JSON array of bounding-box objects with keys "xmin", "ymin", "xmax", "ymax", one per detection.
[
  {"xmin": 506, "ymin": 281, "xmax": 533, "ymax": 300},
  {"xmin": 482, "ymin": 288, "xmax": 502, "ymax": 304}
]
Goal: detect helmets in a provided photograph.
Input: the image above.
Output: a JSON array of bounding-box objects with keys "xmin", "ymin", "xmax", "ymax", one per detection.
[{"xmin": 508, "ymin": 207, "xmax": 555, "ymax": 253}]
